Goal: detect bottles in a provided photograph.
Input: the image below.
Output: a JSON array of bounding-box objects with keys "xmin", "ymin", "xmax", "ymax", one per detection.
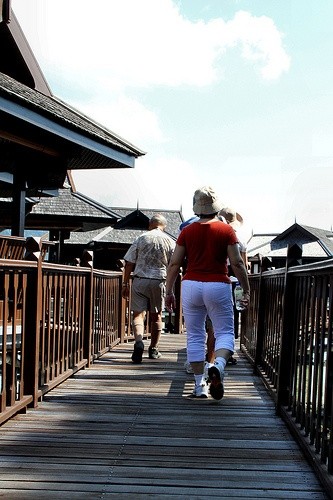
[{"xmin": 234, "ymin": 283, "xmax": 245, "ymax": 310}]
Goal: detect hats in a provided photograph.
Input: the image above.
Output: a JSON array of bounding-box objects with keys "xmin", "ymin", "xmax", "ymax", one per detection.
[
  {"xmin": 193, "ymin": 186, "xmax": 223, "ymax": 214},
  {"xmin": 218, "ymin": 207, "xmax": 244, "ymax": 233}
]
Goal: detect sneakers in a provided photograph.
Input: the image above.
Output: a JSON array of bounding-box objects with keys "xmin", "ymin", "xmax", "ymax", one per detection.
[
  {"xmin": 132, "ymin": 341, "xmax": 144, "ymax": 361},
  {"xmin": 193, "ymin": 382, "xmax": 208, "ymax": 398},
  {"xmin": 208, "ymin": 366, "xmax": 224, "ymax": 400},
  {"xmin": 184, "ymin": 361, "xmax": 194, "ymax": 373},
  {"xmin": 205, "ymin": 363, "xmax": 214, "ymax": 380},
  {"xmin": 148, "ymin": 346, "xmax": 161, "ymax": 359},
  {"xmin": 227, "ymin": 357, "xmax": 237, "ymax": 364}
]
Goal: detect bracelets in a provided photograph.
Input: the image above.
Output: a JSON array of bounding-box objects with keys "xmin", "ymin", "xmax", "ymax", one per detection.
[
  {"xmin": 165, "ymin": 291, "xmax": 174, "ymax": 296},
  {"xmin": 121, "ymin": 281, "xmax": 128, "ymax": 286}
]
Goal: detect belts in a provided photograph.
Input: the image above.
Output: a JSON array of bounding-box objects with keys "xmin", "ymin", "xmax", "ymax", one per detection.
[{"xmin": 134, "ymin": 275, "xmax": 159, "ymax": 280}]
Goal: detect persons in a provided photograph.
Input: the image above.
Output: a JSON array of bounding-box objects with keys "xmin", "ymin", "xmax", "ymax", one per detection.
[
  {"xmin": 166, "ymin": 185, "xmax": 252, "ymax": 401},
  {"xmin": 120, "ymin": 213, "xmax": 177, "ymax": 364}
]
